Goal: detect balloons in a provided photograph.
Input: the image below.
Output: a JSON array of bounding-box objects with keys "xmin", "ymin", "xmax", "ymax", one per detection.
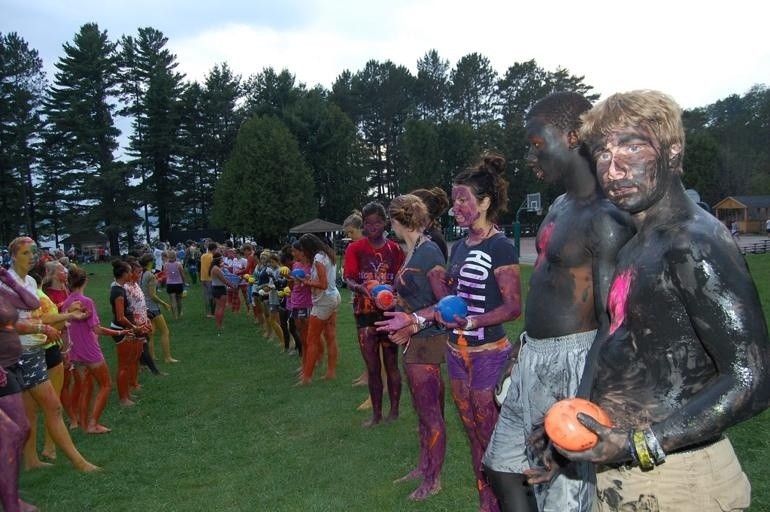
[{"xmin": 545, "ymin": 398, "xmax": 612, "ymax": 451}]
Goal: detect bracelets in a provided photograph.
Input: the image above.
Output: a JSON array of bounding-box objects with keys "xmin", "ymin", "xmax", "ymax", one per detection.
[
  {"xmin": 634, "ymin": 432, "xmax": 654, "ymax": 472},
  {"xmin": 643, "ymin": 427, "xmax": 665, "ymax": 465},
  {"xmin": 628, "ymin": 426, "xmax": 638, "ymax": 467}
]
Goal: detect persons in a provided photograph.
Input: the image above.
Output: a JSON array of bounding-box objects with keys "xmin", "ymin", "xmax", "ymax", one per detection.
[
  {"xmin": 479, "ymin": 93, "xmax": 638, "ymax": 511},
  {"xmin": 152, "ymin": 240, "xmax": 202, "ymax": 320},
  {"xmin": 723, "ymin": 215, "xmax": 733, "ymax": 230},
  {"xmin": 342, "ymin": 151, "xmax": 520, "ymax": 511},
  {"xmin": 111, "ymin": 241, "xmax": 178, "ymax": 406},
  {"xmin": 200, "ymin": 233, "xmax": 341, "ymax": 385},
  {"xmin": 547, "ymin": 92, "xmax": 769, "ymax": 511},
  {"xmin": 765, "ymin": 218, "xmax": 770, "ymax": 238},
  {"xmin": 730, "ymin": 219, "xmax": 742, "ymax": 240},
  {"xmin": 1, "ymin": 236, "xmax": 134, "ymax": 511}
]
